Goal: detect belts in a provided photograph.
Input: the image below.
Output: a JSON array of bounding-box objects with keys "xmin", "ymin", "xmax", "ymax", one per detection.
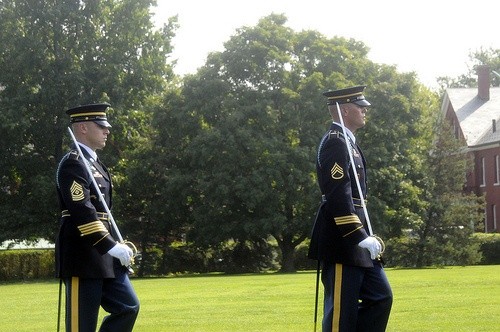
[
  {"xmin": 61, "ymin": 209, "xmax": 110, "ymax": 222},
  {"xmin": 321, "ymin": 194, "xmax": 367, "ymax": 208}
]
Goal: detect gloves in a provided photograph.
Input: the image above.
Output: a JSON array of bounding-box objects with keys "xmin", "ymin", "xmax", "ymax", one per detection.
[
  {"xmin": 107, "ymin": 242, "xmax": 133, "ymax": 267},
  {"xmin": 358, "ymin": 236, "xmax": 382, "ymax": 260}
]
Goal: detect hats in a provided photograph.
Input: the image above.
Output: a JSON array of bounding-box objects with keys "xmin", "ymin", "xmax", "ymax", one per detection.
[
  {"xmin": 323, "ymin": 86, "xmax": 371, "ymax": 106},
  {"xmin": 65, "ymin": 103, "xmax": 113, "ymax": 128}
]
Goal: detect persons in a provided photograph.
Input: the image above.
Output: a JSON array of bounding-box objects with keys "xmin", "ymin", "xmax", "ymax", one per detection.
[
  {"xmin": 307, "ymin": 85, "xmax": 393, "ymax": 332},
  {"xmin": 56, "ymin": 102, "xmax": 142, "ymax": 332}
]
